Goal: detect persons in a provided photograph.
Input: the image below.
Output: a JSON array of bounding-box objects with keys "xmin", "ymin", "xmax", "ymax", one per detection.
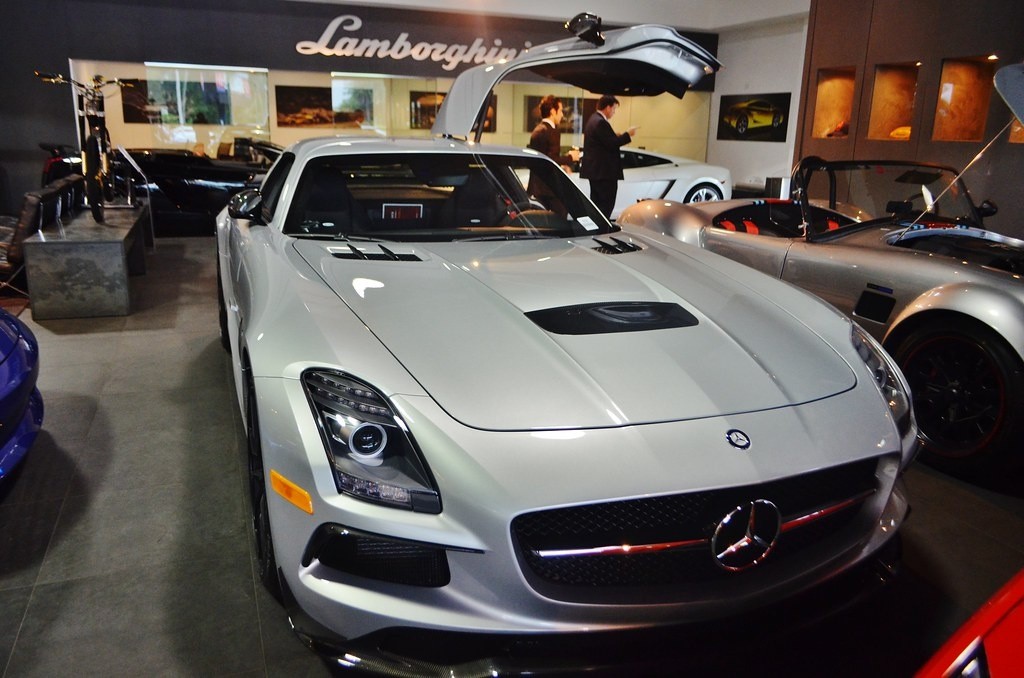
[
  {"xmin": 580, "ymin": 95, "xmax": 635, "ymax": 222},
  {"xmin": 526, "ymin": 94, "xmax": 580, "ymax": 219}
]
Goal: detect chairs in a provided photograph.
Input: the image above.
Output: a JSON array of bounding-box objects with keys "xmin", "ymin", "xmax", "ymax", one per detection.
[{"xmin": 295, "ymin": 164, "xmax": 544, "ymax": 232}]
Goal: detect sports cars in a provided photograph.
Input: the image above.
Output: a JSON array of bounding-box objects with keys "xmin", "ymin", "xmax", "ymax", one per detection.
[
  {"xmin": 544, "ymin": 143, "xmax": 733, "ymax": 231},
  {"xmin": 212, "ymin": 12, "xmax": 918, "ymax": 678},
  {"xmin": 619, "ymin": 59, "xmax": 1024, "ymax": 476},
  {"xmin": 0, "ymin": 307, "xmax": 45, "ymax": 507},
  {"xmin": 112, "ymin": 137, "xmax": 287, "ymax": 234}
]
